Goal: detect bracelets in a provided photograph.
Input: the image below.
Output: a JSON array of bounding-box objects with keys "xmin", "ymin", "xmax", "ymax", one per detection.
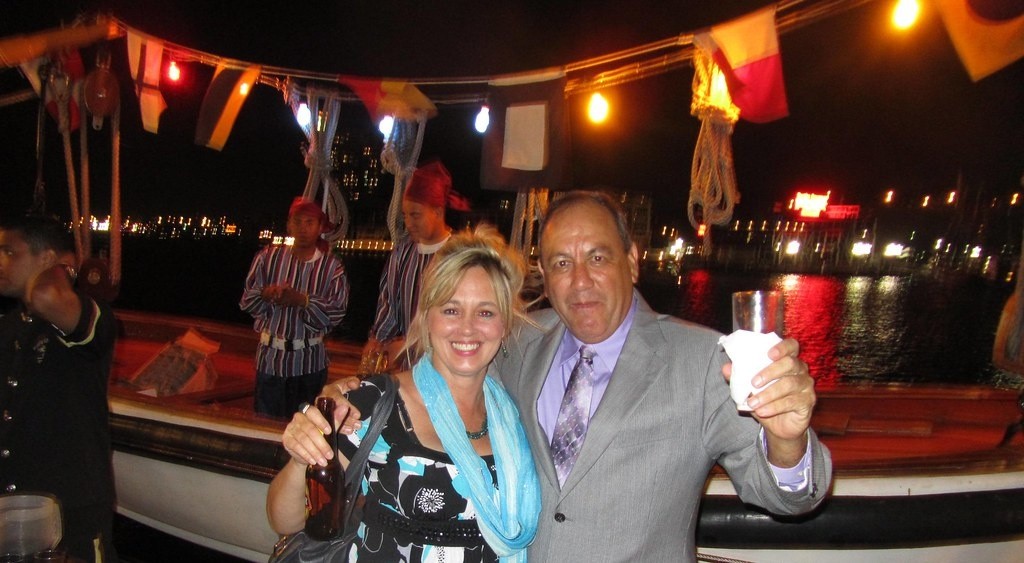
[
  {"xmin": 305, "ymin": 293, "xmax": 308, "ymax": 307},
  {"xmin": 61, "ymin": 263, "xmax": 77, "ymax": 280}
]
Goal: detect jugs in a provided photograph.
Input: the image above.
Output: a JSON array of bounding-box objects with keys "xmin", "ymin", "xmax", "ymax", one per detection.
[{"xmin": 0, "ymin": 495, "xmax": 62, "ymax": 554}]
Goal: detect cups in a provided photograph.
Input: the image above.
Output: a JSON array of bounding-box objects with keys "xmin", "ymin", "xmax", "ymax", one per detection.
[
  {"xmin": 731, "ymin": 290, "xmax": 783, "ymax": 336},
  {"xmin": 34, "ymin": 549, "xmax": 67, "ymax": 563}
]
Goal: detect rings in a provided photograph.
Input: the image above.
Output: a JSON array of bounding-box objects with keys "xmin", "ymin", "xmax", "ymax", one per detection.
[{"xmin": 298, "ymin": 402, "xmax": 310, "ymax": 413}]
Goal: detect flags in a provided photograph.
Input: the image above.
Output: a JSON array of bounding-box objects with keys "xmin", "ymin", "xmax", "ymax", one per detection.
[
  {"xmin": 341, "ymin": 78, "xmax": 437, "ymax": 125},
  {"xmin": 698, "ymin": 10, "xmax": 788, "ymax": 122},
  {"xmin": 24, "ymin": 48, "xmax": 85, "ymax": 131},
  {"xmin": 195, "ymin": 66, "xmax": 261, "ymax": 150},
  {"xmin": 480, "ymin": 70, "xmax": 571, "ymax": 191},
  {"xmin": 126, "ymin": 32, "xmax": 166, "ymax": 135},
  {"xmin": 934, "ymin": 0, "xmax": 1024, "ymax": 83}
]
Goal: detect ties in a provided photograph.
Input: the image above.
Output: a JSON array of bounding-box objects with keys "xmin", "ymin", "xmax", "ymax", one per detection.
[{"xmin": 547, "ymin": 346, "xmax": 600, "ymax": 489}]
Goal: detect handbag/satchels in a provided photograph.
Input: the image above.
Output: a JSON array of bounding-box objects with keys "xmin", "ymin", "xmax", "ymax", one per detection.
[{"xmin": 269, "ymin": 372, "xmax": 399, "ymax": 563}]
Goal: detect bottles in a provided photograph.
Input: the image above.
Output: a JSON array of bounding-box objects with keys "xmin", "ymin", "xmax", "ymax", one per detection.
[{"xmin": 305, "ymin": 397, "xmax": 345, "ymax": 541}]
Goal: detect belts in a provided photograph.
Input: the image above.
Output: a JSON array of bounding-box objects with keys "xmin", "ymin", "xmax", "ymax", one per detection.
[{"xmin": 260, "ymin": 332, "xmax": 323, "ymax": 350}]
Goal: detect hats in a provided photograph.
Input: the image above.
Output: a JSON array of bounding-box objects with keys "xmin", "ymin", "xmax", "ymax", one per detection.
[
  {"xmin": 289, "ymin": 198, "xmax": 326, "ymax": 220},
  {"xmin": 404, "ymin": 161, "xmax": 471, "ymax": 212}
]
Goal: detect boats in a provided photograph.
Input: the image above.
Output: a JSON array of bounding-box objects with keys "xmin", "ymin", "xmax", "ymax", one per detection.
[{"xmin": 105, "ymin": 307, "xmax": 1024, "ymax": 563}]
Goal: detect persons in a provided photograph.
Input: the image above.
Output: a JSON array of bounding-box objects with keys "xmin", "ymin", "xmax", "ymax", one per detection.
[
  {"xmin": 986, "ymin": 248, "xmax": 1012, "ymax": 280},
  {"xmin": 0, "ymin": 214, "xmax": 117, "ymax": 563},
  {"xmin": 316, "ymin": 194, "xmax": 835, "ymax": 563},
  {"xmin": 241, "ymin": 198, "xmax": 349, "ymax": 415},
  {"xmin": 261, "ymin": 225, "xmax": 543, "ymax": 563},
  {"xmin": 990, "ymin": 253, "xmax": 1024, "ymax": 389},
  {"xmin": 363, "ymin": 165, "xmax": 451, "ymax": 371}
]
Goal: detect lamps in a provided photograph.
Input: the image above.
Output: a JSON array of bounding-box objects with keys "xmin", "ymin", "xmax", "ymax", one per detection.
[{"xmin": 83, "ymin": 46, "xmax": 120, "ymax": 118}]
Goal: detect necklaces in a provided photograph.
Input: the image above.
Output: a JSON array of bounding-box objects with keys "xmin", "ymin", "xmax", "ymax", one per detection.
[{"xmin": 468, "ymin": 414, "xmax": 489, "ymax": 439}]
